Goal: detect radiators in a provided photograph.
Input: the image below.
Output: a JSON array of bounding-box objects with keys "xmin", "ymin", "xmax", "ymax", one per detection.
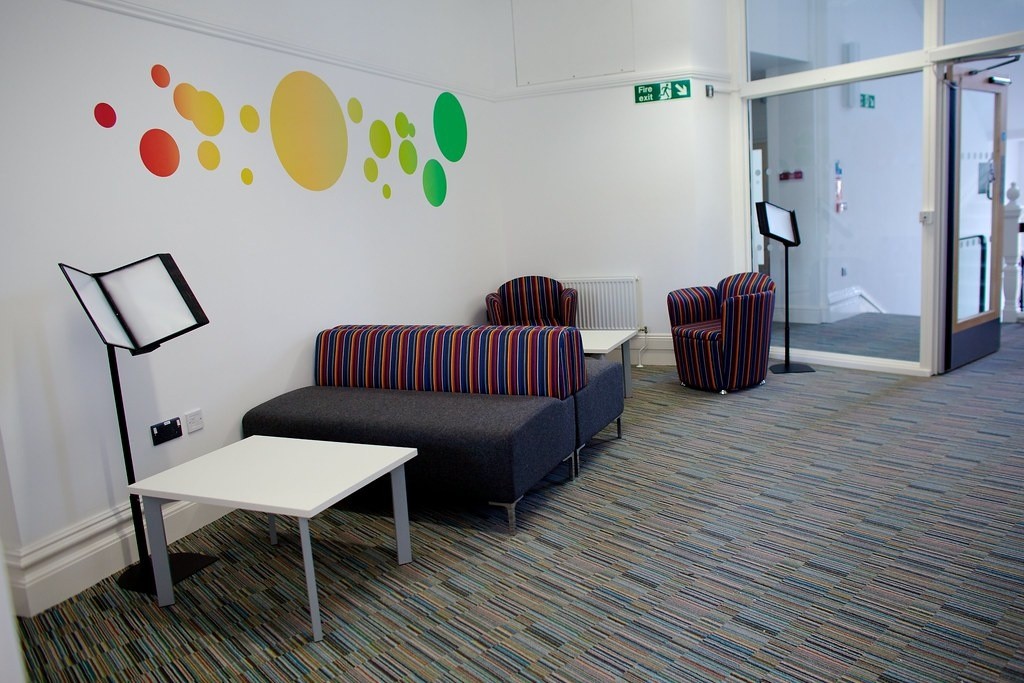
[{"xmin": 554, "ymin": 275, "xmax": 648, "ymax": 334}]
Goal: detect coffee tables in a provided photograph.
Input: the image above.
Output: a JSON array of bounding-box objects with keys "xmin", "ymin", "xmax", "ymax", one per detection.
[
  {"xmin": 126, "ymin": 436, "xmax": 419, "ymax": 641},
  {"xmin": 578, "ymin": 330, "xmax": 639, "ymax": 398}
]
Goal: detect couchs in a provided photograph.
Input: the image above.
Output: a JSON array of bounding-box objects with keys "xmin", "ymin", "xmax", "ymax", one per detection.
[
  {"xmin": 666, "ymin": 272, "xmax": 777, "ymax": 394},
  {"xmin": 484, "ymin": 275, "xmax": 578, "ymax": 330}
]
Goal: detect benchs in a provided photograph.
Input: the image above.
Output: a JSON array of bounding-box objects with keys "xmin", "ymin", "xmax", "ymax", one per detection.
[{"xmin": 241, "ymin": 323, "xmax": 628, "ymax": 531}]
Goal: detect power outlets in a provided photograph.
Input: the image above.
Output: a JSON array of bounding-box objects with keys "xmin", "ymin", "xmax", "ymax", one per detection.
[
  {"xmin": 186, "ymin": 408, "xmax": 205, "ymax": 434},
  {"xmin": 150, "ymin": 416, "xmax": 182, "ymax": 446}
]
